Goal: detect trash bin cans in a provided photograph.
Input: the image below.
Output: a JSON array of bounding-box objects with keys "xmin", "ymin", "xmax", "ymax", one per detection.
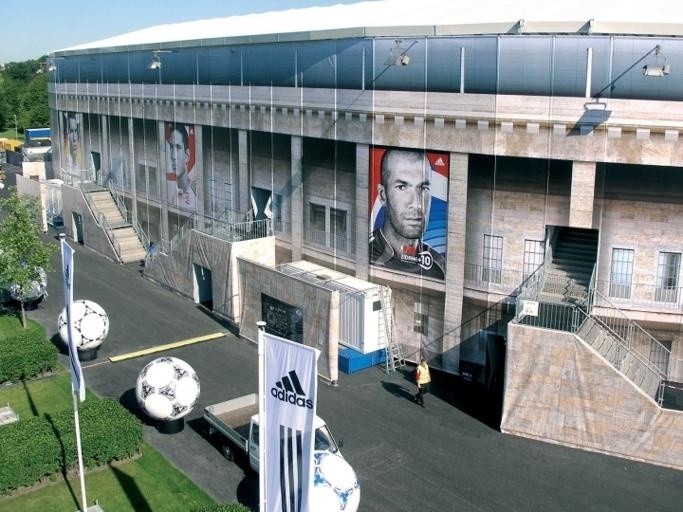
[
  {"xmin": 54, "ymin": 217, "xmax": 63, "ymax": 229},
  {"xmin": 655, "ymin": 380, "xmax": 683, "ymax": 411}
]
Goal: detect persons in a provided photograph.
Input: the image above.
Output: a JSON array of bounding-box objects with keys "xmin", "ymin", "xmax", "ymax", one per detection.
[
  {"xmin": 416, "ymin": 359, "xmax": 431, "ymax": 408},
  {"xmin": 67, "ymin": 113, "xmax": 81, "ymax": 174},
  {"xmin": 168, "ymin": 123, "xmax": 196, "ymax": 211},
  {"xmin": 148, "ymin": 242, "xmax": 157, "ymax": 261},
  {"xmin": 368, "ymin": 147, "xmax": 446, "ymax": 281}
]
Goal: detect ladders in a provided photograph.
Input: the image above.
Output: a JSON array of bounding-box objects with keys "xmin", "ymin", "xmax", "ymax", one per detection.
[{"xmin": 379, "ymin": 284, "xmax": 407, "ymax": 374}]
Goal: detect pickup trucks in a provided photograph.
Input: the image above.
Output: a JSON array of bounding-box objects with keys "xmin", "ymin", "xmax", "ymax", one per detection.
[{"xmin": 203, "ymin": 393, "xmax": 344, "ymax": 475}]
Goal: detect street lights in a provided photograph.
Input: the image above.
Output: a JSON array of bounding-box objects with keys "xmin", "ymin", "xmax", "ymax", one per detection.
[{"xmin": 13, "ymin": 114, "xmax": 17, "ymax": 140}]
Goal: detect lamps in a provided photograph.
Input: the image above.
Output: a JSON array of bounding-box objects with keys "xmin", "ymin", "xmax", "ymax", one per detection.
[
  {"xmin": 639, "ymin": 43, "xmax": 670, "ymax": 81},
  {"xmin": 35, "ymin": 57, "xmax": 60, "ymax": 76},
  {"xmin": 383, "ymin": 38, "xmax": 409, "ymax": 71},
  {"xmin": 145, "ymin": 48, "xmax": 173, "ymax": 71}
]
